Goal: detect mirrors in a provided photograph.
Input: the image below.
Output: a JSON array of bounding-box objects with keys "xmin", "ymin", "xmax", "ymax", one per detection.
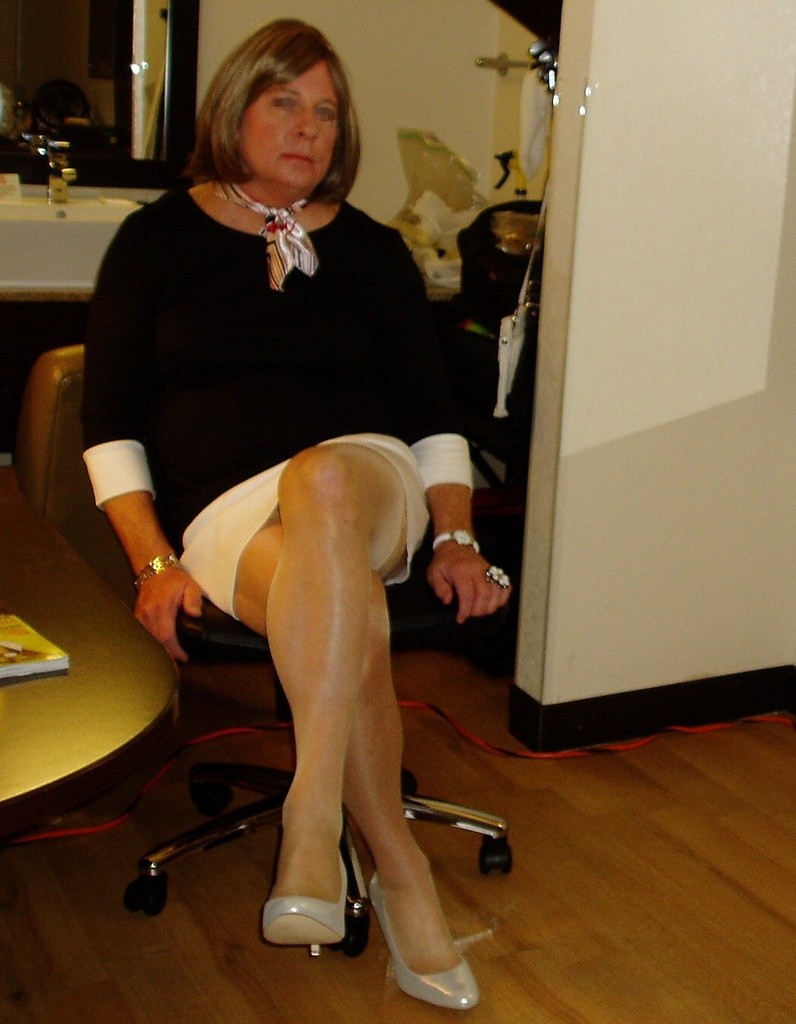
[{"xmin": 0, "ymin": 0, "xmax": 199, "ymax": 192}]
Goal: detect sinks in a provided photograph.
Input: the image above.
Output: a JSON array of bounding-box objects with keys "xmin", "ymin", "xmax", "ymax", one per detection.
[{"xmin": 2, "ymin": 197, "xmax": 144, "ymax": 286}]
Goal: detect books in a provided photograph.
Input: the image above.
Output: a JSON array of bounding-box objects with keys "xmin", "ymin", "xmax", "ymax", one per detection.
[{"xmin": 0, "ymin": 614, "xmax": 71, "ymax": 679}]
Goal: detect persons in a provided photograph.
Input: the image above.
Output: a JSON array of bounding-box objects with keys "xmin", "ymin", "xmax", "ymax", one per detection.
[{"xmin": 79, "ymin": 19, "xmax": 516, "ymax": 1012}]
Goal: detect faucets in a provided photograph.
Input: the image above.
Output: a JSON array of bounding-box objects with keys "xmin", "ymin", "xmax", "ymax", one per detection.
[
  {"xmin": 47, "ymin": 138, "xmax": 77, "ymax": 202},
  {"xmin": 18, "ymin": 133, "xmax": 46, "ymax": 184}
]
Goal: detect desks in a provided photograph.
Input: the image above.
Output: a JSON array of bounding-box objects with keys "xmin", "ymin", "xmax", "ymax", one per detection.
[{"xmin": 0, "ymin": 462, "xmax": 185, "ymax": 808}]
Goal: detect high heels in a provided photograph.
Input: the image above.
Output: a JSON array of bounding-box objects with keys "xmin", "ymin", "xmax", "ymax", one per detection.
[
  {"xmin": 368, "ymin": 872, "xmax": 479, "ymax": 1010},
  {"xmin": 261, "ymin": 848, "xmax": 347, "ymax": 957}
]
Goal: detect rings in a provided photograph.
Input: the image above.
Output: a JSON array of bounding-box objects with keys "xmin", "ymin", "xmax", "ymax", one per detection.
[{"xmin": 485, "ymin": 565, "xmax": 511, "ymax": 590}]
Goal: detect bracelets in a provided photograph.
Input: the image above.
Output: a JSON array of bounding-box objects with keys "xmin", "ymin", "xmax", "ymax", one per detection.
[
  {"xmin": 134, "ymin": 553, "xmax": 183, "ymax": 591},
  {"xmin": 432, "ymin": 529, "xmax": 481, "ymax": 555}
]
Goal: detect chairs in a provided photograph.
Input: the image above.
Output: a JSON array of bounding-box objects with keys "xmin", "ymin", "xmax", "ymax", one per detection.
[{"xmin": 13, "ymin": 343, "xmax": 140, "ymax": 618}]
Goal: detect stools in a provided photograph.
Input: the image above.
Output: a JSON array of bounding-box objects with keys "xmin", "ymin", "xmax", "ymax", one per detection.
[{"xmin": 125, "ymin": 574, "xmax": 515, "ymax": 959}]
